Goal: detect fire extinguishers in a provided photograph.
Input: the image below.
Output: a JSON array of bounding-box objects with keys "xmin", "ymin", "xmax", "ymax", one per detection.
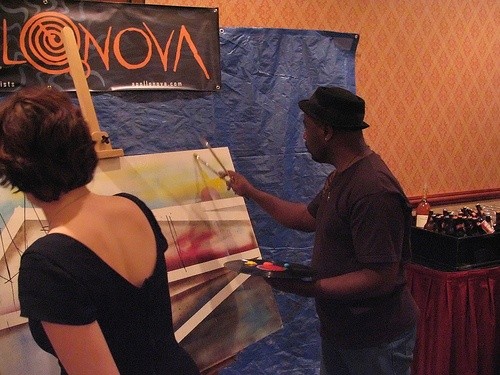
[{"xmin": 416, "ymin": 199, "xmax": 430, "ymax": 229}]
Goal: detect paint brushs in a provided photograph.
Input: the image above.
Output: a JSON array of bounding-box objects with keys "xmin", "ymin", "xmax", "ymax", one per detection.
[{"xmin": 193, "ymin": 138, "xmax": 250, "ymax": 203}]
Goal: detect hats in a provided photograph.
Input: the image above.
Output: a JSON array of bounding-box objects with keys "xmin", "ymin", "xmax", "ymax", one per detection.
[{"xmin": 298, "ymin": 86, "xmax": 369, "ymax": 129}]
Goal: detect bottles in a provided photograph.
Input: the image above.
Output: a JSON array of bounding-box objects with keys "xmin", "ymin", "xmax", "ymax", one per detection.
[{"xmin": 416, "ymin": 187, "xmax": 500, "ymax": 236}]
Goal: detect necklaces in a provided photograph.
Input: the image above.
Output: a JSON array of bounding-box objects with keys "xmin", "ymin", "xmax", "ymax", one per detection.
[{"xmin": 328, "ymin": 145, "xmax": 370, "ymax": 198}]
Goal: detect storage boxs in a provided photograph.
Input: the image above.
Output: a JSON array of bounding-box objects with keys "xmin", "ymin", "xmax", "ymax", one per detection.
[{"xmin": 411, "ymin": 225, "xmax": 500, "ymax": 272}]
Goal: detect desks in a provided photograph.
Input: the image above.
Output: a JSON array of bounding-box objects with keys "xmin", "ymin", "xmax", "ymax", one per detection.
[{"xmin": 405, "ymin": 263, "xmax": 500, "ymax": 375}]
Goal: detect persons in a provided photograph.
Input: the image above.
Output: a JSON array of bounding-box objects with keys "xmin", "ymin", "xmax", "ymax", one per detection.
[
  {"xmin": 219, "ymin": 86, "xmax": 420, "ymax": 375},
  {"xmin": 0, "ymin": 82, "xmax": 201, "ymax": 375}
]
